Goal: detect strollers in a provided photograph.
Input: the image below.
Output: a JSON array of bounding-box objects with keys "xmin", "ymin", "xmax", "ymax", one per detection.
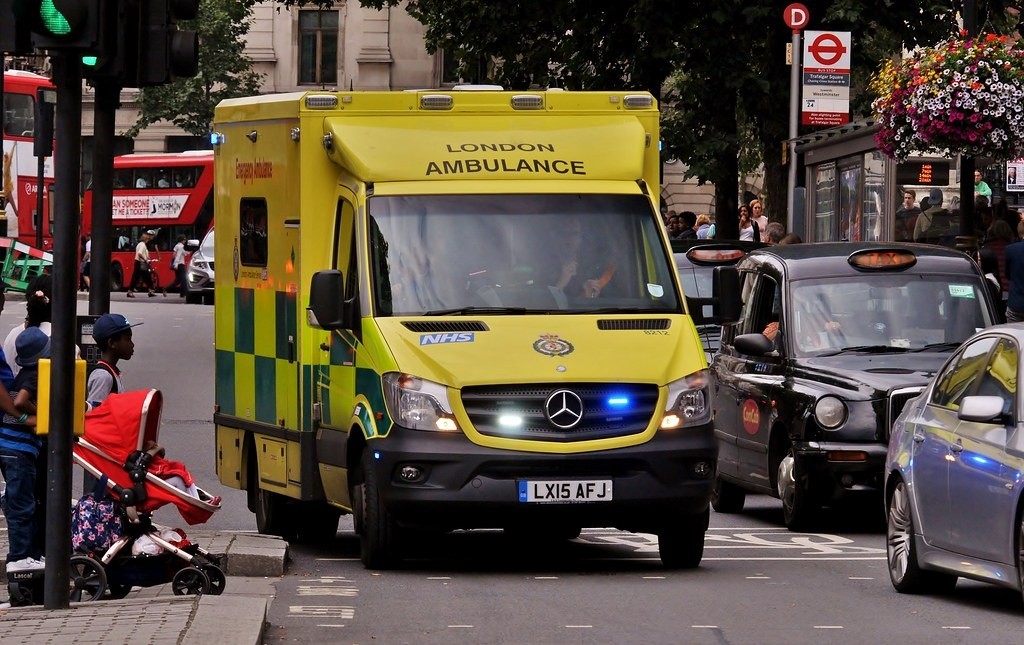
[{"xmin": 68, "ymin": 386, "xmax": 229, "ymax": 595}]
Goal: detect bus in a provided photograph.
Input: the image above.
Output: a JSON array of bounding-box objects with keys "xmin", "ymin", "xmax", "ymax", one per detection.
[
  {"xmin": 3, "ymin": 68, "xmax": 57, "ymax": 275},
  {"xmin": 78, "ymin": 147, "xmax": 216, "ymax": 292}
]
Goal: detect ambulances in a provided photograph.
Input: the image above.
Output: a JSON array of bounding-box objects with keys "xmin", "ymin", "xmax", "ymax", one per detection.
[{"xmin": 209, "ymin": 79, "xmax": 722, "ymax": 567}]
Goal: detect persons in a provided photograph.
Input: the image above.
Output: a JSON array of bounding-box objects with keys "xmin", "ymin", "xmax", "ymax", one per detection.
[
  {"xmin": 0, "ymin": 274, "xmax": 82, "ymax": 563},
  {"xmin": 145, "ymin": 437, "xmax": 222, "ymax": 506},
  {"xmin": 164, "ymin": 233, "xmax": 190, "ymax": 297},
  {"xmin": 532, "ymin": 208, "xmax": 617, "ymax": 300},
  {"xmin": 136, "ymin": 172, "xmax": 148, "ymax": 189},
  {"xmin": 175, "ymin": 173, "xmax": 185, "ymax": 187},
  {"xmin": 896, "ymin": 166, "xmax": 1024, "ymax": 324},
  {"xmin": 157, "ymin": 172, "xmax": 171, "ymax": 188},
  {"xmin": 1008, "ymin": 168, "xmax": 1016, "ymax": 183},
  {"xmin": 659, "ymin": 194, "xmax": 803, "ymax": 248},
  {"xmin": 82, "ymin": 313, "xmax": 144, "ymax": 497},
  {"xmin": 112, "ymin": 171, "xmax": 126, "ymax": 189},
  {"xmin": 80, "ymin": 231, "xmax": 92, "ymax": 293},
  {"xmin": 125, "ymin": 229, "xmax": 160, "ymax": 298},
  {"xmin": 2, "ymin": 140, "xmax": 19, "ymax": 219}
]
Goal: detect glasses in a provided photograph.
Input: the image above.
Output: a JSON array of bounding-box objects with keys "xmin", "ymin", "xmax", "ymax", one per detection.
[
  {"xmin": 754, "ymin": 207, "xmax": 761, "ymax": 209},
  {"xmin": 740, "ymin": 210, "xmax": 747, "ymax": 212}
]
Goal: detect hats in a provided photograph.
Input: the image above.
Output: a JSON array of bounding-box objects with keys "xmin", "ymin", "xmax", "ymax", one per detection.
[
  {"xmin": 92, "ymin": 312, "xmax": 144, "ymax": 343},
  {"xmin": 919, "ymin": 197, "xmax": 931, "ymax": 210},
  {"xmin": 14, "ymin": 326, "xmax": 54, "ymax": 368},
  {"xmin": 904, "ymin": 190, "xmax": 916, "ymax": 198},
  {"xmin": 927, "ymin": 189, "xmax": 943, "ymax": 205},
  {"xmin": 139, "ymin": 233, "xmax": 151, "ymax": 241}
]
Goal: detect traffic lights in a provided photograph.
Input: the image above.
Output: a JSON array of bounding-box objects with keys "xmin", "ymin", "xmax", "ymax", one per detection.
[{"xmin": 0, "ymin": 0, "xmax": 109, "ymax": 57}]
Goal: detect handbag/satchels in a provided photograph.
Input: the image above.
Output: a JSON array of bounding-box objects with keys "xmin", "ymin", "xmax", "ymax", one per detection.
[
  {"xmin": 70, "ymin": 473, "xmax": 124, "ymax": 557},
  {"xmin": 170, "ymin": 257, "xmax": 182, "ymax": 271},
  {"xmin": 123, "ymin": 243, "xmax": 133, "ymax": 251}
]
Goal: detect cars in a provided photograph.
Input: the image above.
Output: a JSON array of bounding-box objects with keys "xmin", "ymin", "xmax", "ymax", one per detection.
[
  {"xmin": 182, "ymin": 226, "xmax": 215, "ymax": 306},
  {"xmin": 883, "ymin": 322, "xmax": 1024, "ymax": 610},
  {"xmin": 659, "ymin": 237, "xmax": 850, "ymax": 376},
  {"xmin": 705, "ymin": 240, "xmax": 1000, "ymax": 533}
]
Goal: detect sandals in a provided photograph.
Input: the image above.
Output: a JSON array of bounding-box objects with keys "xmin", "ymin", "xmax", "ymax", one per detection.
[
  {"xmin": 127, "ymin": 292, "xmax": 136, "ymax": 298},
  {"xmin": 148, "ymin": 291, "xmax": 157, "ymax": 297}
]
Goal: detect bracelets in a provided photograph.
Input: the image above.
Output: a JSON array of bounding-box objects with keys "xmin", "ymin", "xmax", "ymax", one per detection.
[{"xmin": 19, "ymin": 413, "xmax": 28, "ymax": 422}]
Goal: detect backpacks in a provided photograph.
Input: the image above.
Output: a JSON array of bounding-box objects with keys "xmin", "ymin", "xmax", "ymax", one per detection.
[{"xmin": 923, "ymin": 211, "xmax": 951, "ymax": 241}]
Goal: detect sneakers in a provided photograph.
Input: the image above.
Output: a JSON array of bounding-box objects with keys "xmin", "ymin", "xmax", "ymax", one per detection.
[{"xmin": 6, "ymin": 555, "xmax": 46, "ymax": 572}]
